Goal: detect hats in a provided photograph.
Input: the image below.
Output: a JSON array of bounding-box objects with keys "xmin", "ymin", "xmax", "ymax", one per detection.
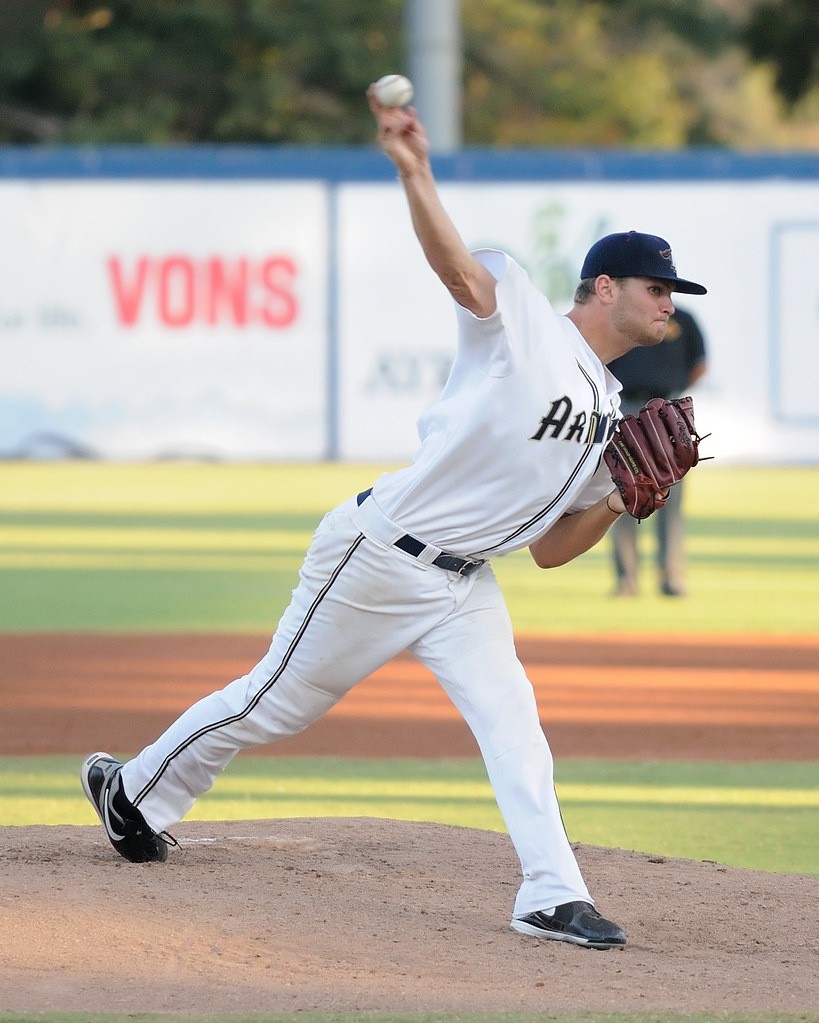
[{"xmin": 581, "ymin": 231, "xmax": 707, "ymax": 296}]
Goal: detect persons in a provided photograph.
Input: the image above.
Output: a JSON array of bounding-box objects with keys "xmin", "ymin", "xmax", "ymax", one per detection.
[
  {"xmin": 606, "ymin": 306, "xmax": 707, "ymax": 597},
  {"xmin": 81, "ymin": 83, "xmax": 708, "ymax": 949}
]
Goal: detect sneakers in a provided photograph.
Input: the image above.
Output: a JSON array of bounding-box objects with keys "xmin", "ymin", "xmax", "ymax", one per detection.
[
  {"xmin": 510, "ymin": 899, "xmax": 625, "ymax": 950},
  {"xmin": 81, "ymin": 751, "xmax": 182, "ymax": 863}
]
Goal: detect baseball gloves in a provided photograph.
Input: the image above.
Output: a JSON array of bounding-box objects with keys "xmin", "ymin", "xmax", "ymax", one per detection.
[{"xmin": 604, "ymin": 395, "xmax": 701, "ymax": 525}]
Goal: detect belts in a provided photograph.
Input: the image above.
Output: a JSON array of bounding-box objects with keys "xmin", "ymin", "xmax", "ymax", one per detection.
[{"xmin": 357, "ymin": 488, "xmax": 488, "ymax": 575}]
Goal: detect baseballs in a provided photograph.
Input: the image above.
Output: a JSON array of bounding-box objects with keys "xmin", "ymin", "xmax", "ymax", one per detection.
[{"xmin": 374, "ymin": 74, "xmax": 413, "ymax": 109}]
